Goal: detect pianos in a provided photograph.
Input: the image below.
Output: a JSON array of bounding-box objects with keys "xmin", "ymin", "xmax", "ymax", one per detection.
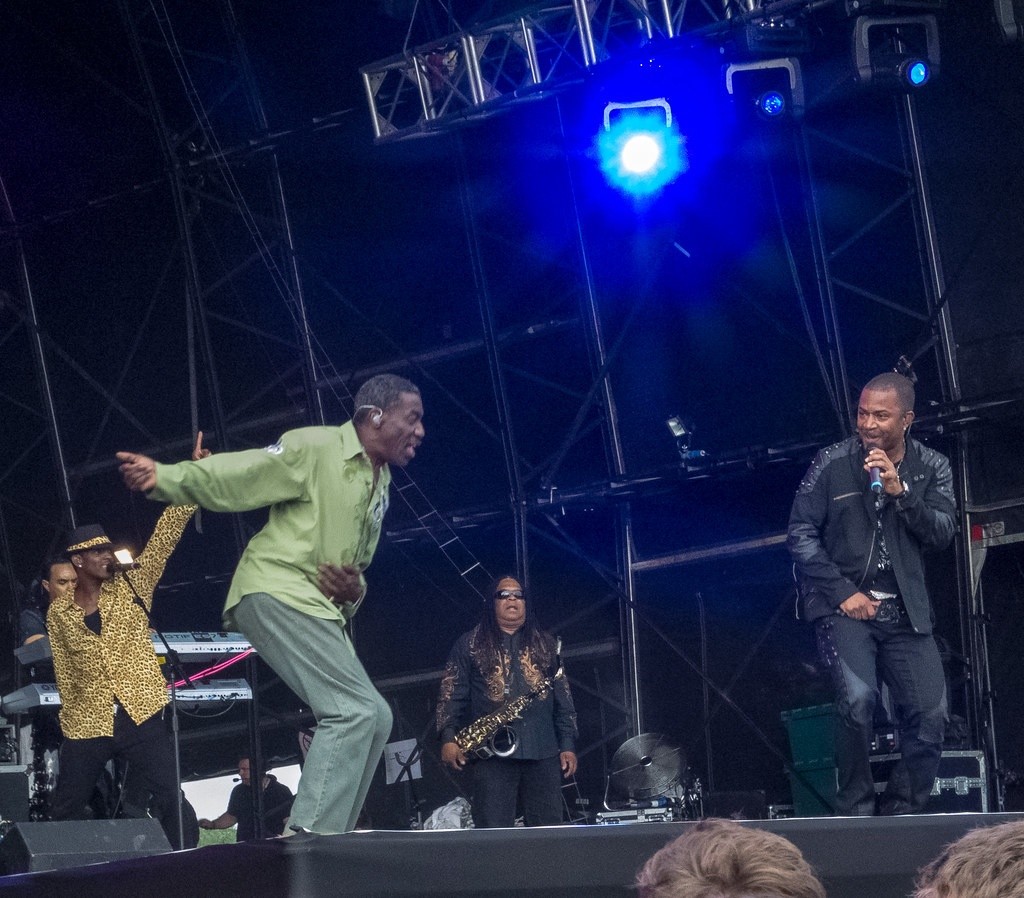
[{"xmin": 2, "ymin": 627, "xmax": 256, "ymax": 722}]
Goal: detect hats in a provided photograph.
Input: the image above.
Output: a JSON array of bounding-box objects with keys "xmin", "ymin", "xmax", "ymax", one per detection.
[{"xmin": 63, "ymin": 524, "xmax": 115, "ymax": 559}]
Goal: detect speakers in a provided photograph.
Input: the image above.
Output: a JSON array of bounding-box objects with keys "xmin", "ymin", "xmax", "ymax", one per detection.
[{"xmin": 0, "ymin": 818, "xmax": 173, "ymax": 875}]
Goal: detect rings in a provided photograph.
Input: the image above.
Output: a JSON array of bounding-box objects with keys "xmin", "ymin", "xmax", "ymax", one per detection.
[{"xmin": 329, "ymin": 596, "xmax": 334, "ymax": 602}]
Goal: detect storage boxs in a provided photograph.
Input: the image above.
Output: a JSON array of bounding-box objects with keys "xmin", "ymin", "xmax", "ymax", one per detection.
[{"xmin": 781, "ymin": 700, "xmax": 988, "ymax": 816}]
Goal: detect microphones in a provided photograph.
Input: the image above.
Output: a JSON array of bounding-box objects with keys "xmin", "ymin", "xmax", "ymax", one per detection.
[
  {"xmin": 107, "ymin": 562, "xmax": 141, "ymax": 573},
  {"xmin": 866, "ymin": 442, "xmax": 882, "ymax": 495},
  {"xmin": 233, "ymin": 778, "xmax": 242, "ymax": 782}
]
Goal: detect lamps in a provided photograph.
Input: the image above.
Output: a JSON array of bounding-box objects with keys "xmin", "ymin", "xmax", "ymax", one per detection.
[{"xmin": 601, "ymin": 0, "xmax": 945, "ymax": 180}]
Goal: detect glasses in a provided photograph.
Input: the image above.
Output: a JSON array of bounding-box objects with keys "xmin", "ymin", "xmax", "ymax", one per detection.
[{"xmin": 494, "ymin": 589, "xmax": 526, "ymax": 599}]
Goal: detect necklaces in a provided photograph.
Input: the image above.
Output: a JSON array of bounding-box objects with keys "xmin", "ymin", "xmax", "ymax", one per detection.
[{"xmin": 896, "ymin": 437, "xmax": 906, "ymax": 474}]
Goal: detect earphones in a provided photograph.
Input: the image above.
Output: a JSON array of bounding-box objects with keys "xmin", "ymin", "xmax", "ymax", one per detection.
[{"xmin": 372, "ymin": 414, "xmax": 381, "ymax": 425}]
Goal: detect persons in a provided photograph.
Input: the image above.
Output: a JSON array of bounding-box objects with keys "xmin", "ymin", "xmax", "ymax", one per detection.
[
  {"xmin": 431, "ymin": 575, "xmax": 580, "ymax": 827},
  {"xmin": 16, "ymin": 558, "xmax": 81, "ymax": 684},
  {"xmin": 197, "ymin": 752, "xmax": 292, "ymax": 843},
  {"xmin": 45, "ymin": 430, "xmax": 213, "ymax": 846},
  {"xmin": 635, "ymin": 818, "xmax": 826, "ymax": 898},
  {"xmin": 784, "ymin": 372, "xmax": 958, "ymax": 817},
  {"xmin": 911, "ymin": 820, "xmax": 1024, "ymax": 898},
  {"xmin": 115, "ymin": 373, "xmax": 426, "ymax": 837}
]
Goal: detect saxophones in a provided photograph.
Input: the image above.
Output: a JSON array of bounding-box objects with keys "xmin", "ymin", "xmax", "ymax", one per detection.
[{"xmin": 456, "ymin": 634, "xmax": 567, "ymax": 764}]
